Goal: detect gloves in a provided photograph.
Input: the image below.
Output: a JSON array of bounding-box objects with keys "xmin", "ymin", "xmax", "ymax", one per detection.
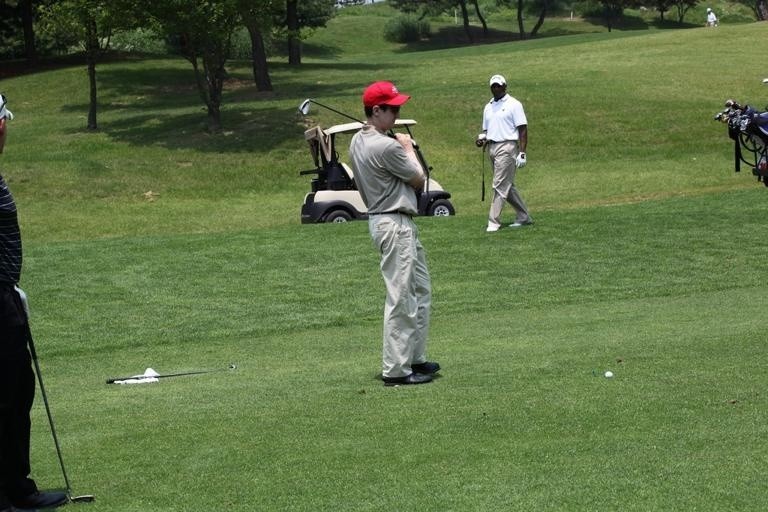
[
  {"xmin": 476, "ymin": 134, "xmax": 486, "ymax": 147},
  {"xmin": 516, "ymin": 152, "xmax": 527, "ymax": 168}
]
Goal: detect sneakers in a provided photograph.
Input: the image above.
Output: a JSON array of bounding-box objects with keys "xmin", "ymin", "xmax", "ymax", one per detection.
[
  {"xmin": 487, "ymin": 221, "xmax": 522, "ymax": 231},
  {"xmin": 383, "ymin": 362, "xmax": 440, "ymax": 385}
]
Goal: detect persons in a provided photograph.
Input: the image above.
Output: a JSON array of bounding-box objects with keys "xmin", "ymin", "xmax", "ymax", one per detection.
[
  {"xmin": 0, "ymin": 95, "xmax": 70, "ymax": 511},
  {"xmin": 705, "ymin": 7, "xmax": 719, "ymax": 26},
  {"xmin": 350, "ymin": 79, "xmax": 440, "ymax": 384},
  {"xmin": 746, "ymin": 77, "xmax": 768, "ymax": 125},
  {"xmin": 476, "ymin": 73, "xmax": 530, "ymax": 233}
]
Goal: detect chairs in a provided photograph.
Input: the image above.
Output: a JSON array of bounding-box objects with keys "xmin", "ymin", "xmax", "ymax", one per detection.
[{"xmin": 339, "ymin": 161, "xmax": 354, "ymax": 181}]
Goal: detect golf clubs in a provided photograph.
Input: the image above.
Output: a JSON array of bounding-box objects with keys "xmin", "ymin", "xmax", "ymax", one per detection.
[
  {"xmin": 105, "ymin": 364, "xmax": 238, "ymax": 385},
  {"xmin": 714, "ymin": 100, "xmax": 746, "ymax": 124},
  {"xmin": 297, "ymin": 97, "xmax": 367, "ymax": 127},
  {"xmin": 25, "ymin": 328, "xmax": 94, "ymax": 502},
  {"xmin": 480, "ymin": 139, "xmax": 496, "ymax": 201}
]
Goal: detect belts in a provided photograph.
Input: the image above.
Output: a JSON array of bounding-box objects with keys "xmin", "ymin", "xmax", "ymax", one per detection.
[{"xmin": 373, "ymin": 211, "xmax": 405, "ymax": 215}]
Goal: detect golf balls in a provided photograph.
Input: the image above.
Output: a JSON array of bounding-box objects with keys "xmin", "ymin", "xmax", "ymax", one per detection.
[{"xmin": 603, "ymin": 371, "xmax": 613, "ymax": 378}]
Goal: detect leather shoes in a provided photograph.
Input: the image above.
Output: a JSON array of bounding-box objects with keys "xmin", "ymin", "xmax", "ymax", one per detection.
[{"xmin": 0, "ymin": 492, "xmax": 68, "ymax": 512}]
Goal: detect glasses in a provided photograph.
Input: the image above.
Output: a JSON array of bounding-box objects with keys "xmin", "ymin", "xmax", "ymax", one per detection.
[{"xmin": 0, "ymin": 95, "xmax": 7, "ymax": 112}]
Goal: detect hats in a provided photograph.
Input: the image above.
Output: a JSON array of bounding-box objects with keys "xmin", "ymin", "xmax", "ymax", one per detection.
[
  {"xmin": 0, "ymin": 95, "xmax": 14, "ymax": 120},
  {"xmin": 363, "ymin": 82, "xmax": 411, "ymax": 106},
  {"xmin": 489, "ymin": 75, "xmax": 506, "ymax": 87}
]
